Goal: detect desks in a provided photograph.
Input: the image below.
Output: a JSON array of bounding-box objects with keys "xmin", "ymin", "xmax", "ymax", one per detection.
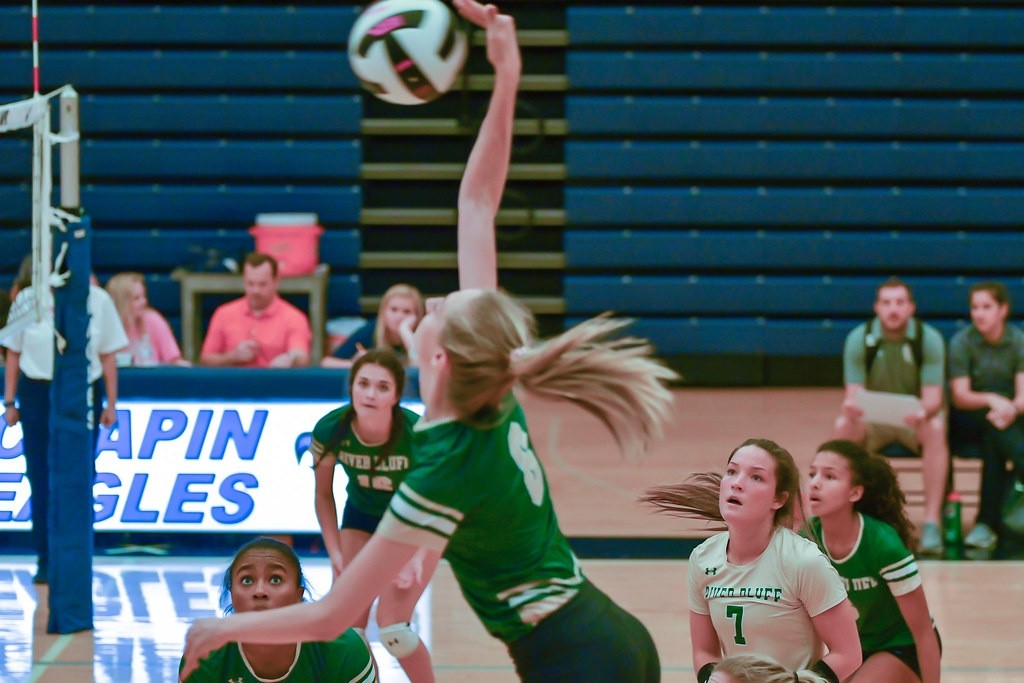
[{"xmin": 172, "ymin": 263, "xmax": 329, "ymax": 365}]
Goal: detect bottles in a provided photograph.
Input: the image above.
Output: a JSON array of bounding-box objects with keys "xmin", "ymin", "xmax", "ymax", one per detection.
[
  {"xmin": 136, "ymin": 333, "xmax": 159, "ymax": 367},
  {"xmin": 944, "ymin": 490, "xmax": 962, "ymax": 558}
]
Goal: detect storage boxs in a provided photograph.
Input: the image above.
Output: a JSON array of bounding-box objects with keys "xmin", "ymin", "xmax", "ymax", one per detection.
[{"xmin": 249, "ymin": 212, "xmax": 323, "ymax": 278}]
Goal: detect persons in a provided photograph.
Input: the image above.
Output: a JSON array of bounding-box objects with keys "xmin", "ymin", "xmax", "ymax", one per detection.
[
  {"xmin": 833, "ymin": 277, "xmax": 948, "ymax": 556},
  {"xmin": 307, "ymin": 350, "xmax": 441, "ymax": 683},
  {"xmin": 0, "ymin": 255, "xmax": 191, "ymax": 587},
  {"xmin": 177, "ymin": 537, "xmax": 375, "ymax": 683},
  {"xmin": 947, "ymin": 283, "xmax": 1024, "ymax": 548},
  {"xmin": 640, "ymin": 437, "xmax": 943, "ymax": 683},
  {"xmin": 320, "ymin": 284, "xmax": 425, "ymax": 369},
  {"xmin": 198, "ymin": 252, "xmax": 312, "ymax": 370},
  {"xmin": 178, "ymin": 0, "xmax": 677, "ymax": 683}
]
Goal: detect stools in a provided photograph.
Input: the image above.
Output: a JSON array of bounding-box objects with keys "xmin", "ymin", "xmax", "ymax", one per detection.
[{"xmin": 875, "ymin": 438, "xmax": 1024, "ymax": 557}]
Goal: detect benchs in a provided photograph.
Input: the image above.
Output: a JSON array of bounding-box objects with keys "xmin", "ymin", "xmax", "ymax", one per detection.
[
  {"xmin": 0, "ymin": 4, "xmax": 361, "ymax": 355},
  {"xmin": 561, "ymin": 8, "xmax": 1024, "ymax": 387}
]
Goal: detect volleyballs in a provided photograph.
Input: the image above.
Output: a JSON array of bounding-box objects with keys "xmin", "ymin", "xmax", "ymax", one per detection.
[{"xmin": 347, "ymin": 0, "xmax": 471, "ymax": 105}]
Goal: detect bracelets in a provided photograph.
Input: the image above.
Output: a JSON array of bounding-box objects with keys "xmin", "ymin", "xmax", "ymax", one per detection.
[{"xmin": 3, "ymin": 401, "xmax": 15, "ymax": 407}]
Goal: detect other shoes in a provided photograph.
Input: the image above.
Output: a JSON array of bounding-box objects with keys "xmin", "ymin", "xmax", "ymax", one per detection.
[
  {"xmin": 963, "ymin": 522, "xmax": 997, "ymax": 548},
  {"xmin": 33, "ymin": 566, "xmax": 47, "ymax": 584},
  {"xmin": 916, "ymin": 522, "xmax": 944, "ymax": 555}
]
